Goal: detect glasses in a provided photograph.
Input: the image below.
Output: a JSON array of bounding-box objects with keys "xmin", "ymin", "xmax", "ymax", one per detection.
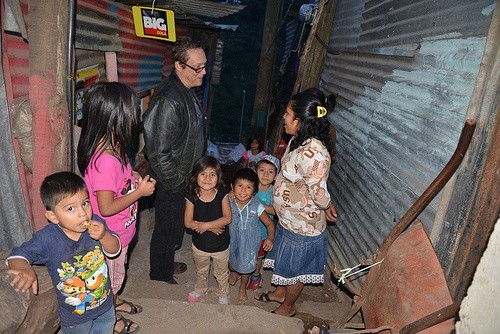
[{"xmin": 179, "ymin": 58, "xmax": 210, "ymax": 73}]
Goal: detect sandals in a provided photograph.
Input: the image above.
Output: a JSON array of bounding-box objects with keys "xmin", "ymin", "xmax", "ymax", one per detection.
[
  {"xmin": 114, "ymin": 299, "xmax": 143, "ymax": 315},
  {"xmin": 114, "ymin": 314, "xmax": 140, "ymax": 334}
]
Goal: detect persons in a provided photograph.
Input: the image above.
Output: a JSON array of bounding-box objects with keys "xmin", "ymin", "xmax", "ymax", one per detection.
[
  {"xmin": 76, "ymin": 81, "xmax": 156, "ymax": 334},
  {"xmin": 141, "ymin": 39, "xmax": 207, "ymax": 285},
  {"xmin": 281, "ymin": 87, "xmax": 337, "ymax": 163},
  {"xmin": 183, "ymin": 135, "xmax": 280, "ymax": 306},
  {"xmin": 5, "ymin": 172, "xmax": 121, "ymax": 334},
  {"xmin": 255, "ymin": 92, "xmax": 338, "ymax": 318}
]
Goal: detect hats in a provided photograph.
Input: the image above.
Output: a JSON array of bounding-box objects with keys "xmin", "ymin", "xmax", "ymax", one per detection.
[{"xmin": 260, "ymin": 154, "xmax": 281, "ymax": 175}]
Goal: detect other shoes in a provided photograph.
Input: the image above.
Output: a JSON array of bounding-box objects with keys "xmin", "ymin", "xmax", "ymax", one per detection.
[
  {"xmin": 173, "ymin": 262, "xmax": 187, "ymax": 274},
  {"xmin": 188, "ymin": 288, "xmax": 208, "ymax": 302},
  {"xmin": 218, "ymin": 295, "xmax": 230, "ymax": 305},
  {"xmin": 167, "ymin": 276, "xmax": 179, "ymax": 285}
]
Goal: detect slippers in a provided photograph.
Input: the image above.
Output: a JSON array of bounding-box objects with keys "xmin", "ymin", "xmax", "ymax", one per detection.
[
  {"xmin": 271, "ymin": 309, "xmax": 296, "ymax": 317},
  {"xmin": 254, "ymin": 291, "xmax": 284, "ymax": 304}
]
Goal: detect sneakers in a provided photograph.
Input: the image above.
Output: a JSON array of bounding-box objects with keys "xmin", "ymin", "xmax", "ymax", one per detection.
[{"xmin": 247, "ymin": 273, "xmax": 263, "ymax": 291}]
